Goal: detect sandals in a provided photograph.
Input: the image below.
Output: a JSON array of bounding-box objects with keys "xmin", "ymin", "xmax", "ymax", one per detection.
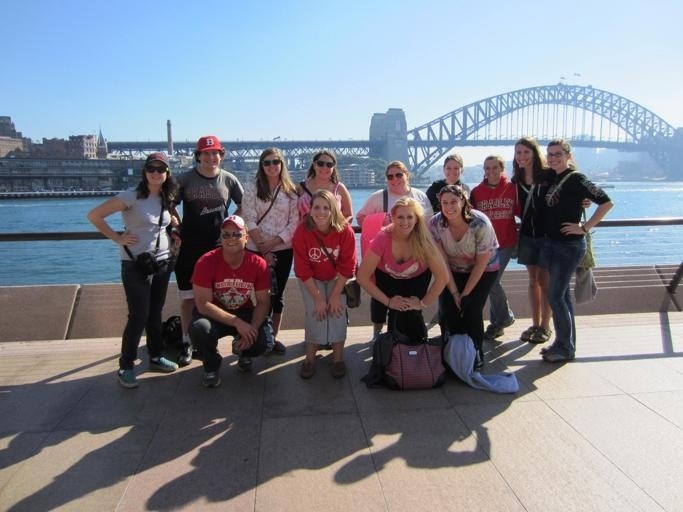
[
  {"xmin": 530, "ymin": 328, "xmax": 553, "ymax": 343},
  {"xmin": 521, "ymin": 325, "xmax": 540, "ymax": 342}
]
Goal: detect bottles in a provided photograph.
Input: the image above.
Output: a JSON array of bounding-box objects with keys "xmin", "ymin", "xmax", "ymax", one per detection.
[
  {"xmin": 233, "ymin": 337, "xmax": 240, "ymax": 361},
  {"xmin": 268, "ymin": 264, "xmax": 278, "ymax": 296}
]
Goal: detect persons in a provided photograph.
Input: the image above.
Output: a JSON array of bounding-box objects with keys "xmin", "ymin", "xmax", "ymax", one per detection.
[
  {"xmin": 240, "ymin": 148, "xmax": 301, "ymax": 355},
  {"xmin": 538, "ymin": 139, "xmax": 615, "ymax": 363},
  {"xmin": 291, "ymin": 188, "xmax": 358, "ymax": 380},
  {"xmin": 187, "ymin": 216, "xmax": 274, "ymax": 386},
  {"xmin": 356, "ymin": 197, "xmax": 448, "ymax": 340},
  {"xmin": 294, "ymin": 149, "xmax": 355, "ymax": 223},
  {"xmin": 173, "ymin": 136, "xmax": 244, "ymax": 367},
  {"xmin": 425, "ymin": 156, "xmax": 472, "ymax": 212},
  {"xmin": 356, "ymin": 160, "xmax": 433, "ymax": 232},
  {"xmin": 468, "ymin": 157, "xmax": 522, "ymax": 340},
  {"xmin": 429, "ymin": 186, "xmax": 500, "ymax": 368},
  {"xmin": 86, "ymin": 151, "xmax": 180, "ymax": 389},
  {"xmin": 508, "ymin": 136, "xmax": 591, "ymax": 343}
]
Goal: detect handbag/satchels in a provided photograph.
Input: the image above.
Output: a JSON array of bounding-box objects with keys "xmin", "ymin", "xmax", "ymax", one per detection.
[
  {"xmin": 308, "ymin": 221, "xmax": 361, "ymax": 310},
  {"xmin": 377, "ymin": 307, "xmax": 446, "ymax": 391},
  {"xmin": 299, "ymin": 179, "xmax": 314, "ymax": 203},
  {"xmin": 510, "ymin": 179, "xmax": 535, "ymax": 259},
  {"xmin": 382, "ymin": 186, "xmax": 391, "ymax": 214},
  {"xmin": 573, "ymin": 267, "xmax": 598, "ymax": 306},
  {"xmin": 118, "ymin": 191, "xmax": 165, "ymax": 276},
  {"xmin": 247, "ymin": 183, "xmax": 283, "ymax": 242},
  {"xmin": 549, "ymin": 170, "xmax": 595, "ymax": 270}
]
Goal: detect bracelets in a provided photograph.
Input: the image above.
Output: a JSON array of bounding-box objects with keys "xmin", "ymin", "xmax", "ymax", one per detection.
[
  {"xmin": 452, "ymin": 291, "xmax": 460, "ymax": 295},
  {"xmin": 388, "ymin": 297, "xmax": 390, "ymax": 308},
  {"xmin": 581, "ymin": 225, "xmax": 586, "ymax": 232}
]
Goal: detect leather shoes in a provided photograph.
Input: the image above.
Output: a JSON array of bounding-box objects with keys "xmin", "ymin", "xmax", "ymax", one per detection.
[
  {"xmin": 177, "ymin": 345, "xmax": 193, "ymax": 366},
  {"xmin": 233, "ymin": 341, "xmax": 243, "ymax": 354}
]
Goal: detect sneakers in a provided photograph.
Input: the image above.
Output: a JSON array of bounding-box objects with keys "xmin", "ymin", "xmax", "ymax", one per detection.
[
  {"xmin": 215, "ymin": 350, "xmax": 222, "ymax": 361},
  {"xmin": 117, "ymin": 367, "xmax": 138, "ymax": 388},
  {"xmin": 149, "ymin": 358, "xmax": 179, "ymax": 372},
  {"xmin": 202, "ymin": 369, "xmax": 221, "ymax": 388},
  {"xmin": 485, "ymin": 324, "xmax": 504, "ymax": 340},
  {"xmin": 542, "ymin": 344, "xmax": 558, "ymax": 352},
  {"xmin": 543, "ymin": 352, "xmax": 571, "ymax": 364},
  {"xmin": 238, "ymin": 353, "xmax": 253, "ymax": 372},
  {"xmin": 301, "ymin": 360, "xmax": 314, "ymax": 379},
  {"xmin": 332, "ymin": 361, "xmax": 345, "ymax": 378},
  {"xmin": 273, "ymin": 341, "xmax": 287, "ymax": 354}
]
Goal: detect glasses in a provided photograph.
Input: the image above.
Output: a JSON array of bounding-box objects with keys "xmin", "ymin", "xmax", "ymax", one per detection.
[
  {"xmin": 387, "ymin": 173, "xmax": 405, "ymax": 180},
  {"xmin": 220, "ymin": 232, "xmax": 244, "ymax": 240},
  {"xmin": 547, "ymin": 150, "xmax": 564, "ymax": 158},
  {"xmin": 260, "ymin": 159, "xmax": 282, "ymax": 166},
  {"xmin": 145, "ymin": 165, "xmax": 167, "ymax": 174},
  {"xmin": 314, "ymin": 160, "xmax": 336, "ymax": 168}
]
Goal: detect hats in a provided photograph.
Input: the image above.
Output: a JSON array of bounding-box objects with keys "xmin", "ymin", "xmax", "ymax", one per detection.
[
  {"xmin": 144, "ymin": 153, "xmax": 169, "ymax": 166},
  {"xmin": 221, "ymin": 216, "xmax": 246, "ymax": 232},
  {"xmin": 197, "ymin": 136, "xmax": 223, "ymax": 153}
]
